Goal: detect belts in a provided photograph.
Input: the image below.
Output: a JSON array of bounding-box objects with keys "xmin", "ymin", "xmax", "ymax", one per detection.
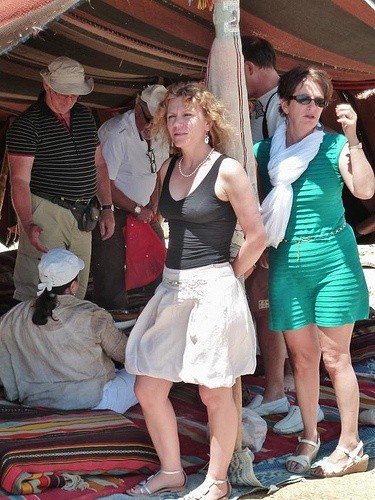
[{"xmin": 30, "ymin": 189, "xmax": 88, "ymax": 207}]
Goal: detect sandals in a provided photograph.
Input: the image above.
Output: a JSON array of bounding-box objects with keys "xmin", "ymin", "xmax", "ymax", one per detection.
[
  {"xmin": 178, "ymin": 473, "xmax": 232, "ymax": 500},
  {"xmin": 126, "ymin": 468, "xmax": 188, "ymax": 497}
]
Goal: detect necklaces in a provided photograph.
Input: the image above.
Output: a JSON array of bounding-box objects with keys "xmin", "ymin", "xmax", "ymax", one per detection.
[{"xmin": 177, "ymin": 147, "xmax": 215, "ymax": 177}]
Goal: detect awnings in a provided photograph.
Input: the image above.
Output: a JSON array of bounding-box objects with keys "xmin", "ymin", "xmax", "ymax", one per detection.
[{"xmin": 0, "ymin": 0, "xmax": 375, "ymax": 142}]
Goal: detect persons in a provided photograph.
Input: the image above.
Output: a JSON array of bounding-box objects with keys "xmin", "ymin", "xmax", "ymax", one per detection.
[
  {"xmin": 243, "ymin": 35, "xmax": 325, "ymax": 434},
  {"xmin": 251, "ymin": 66, "xmax": 374, "ymax": 478},
  {"xmin": 7, "ymin": 59, "xmax": 116, "ymax": 311},
  {"xmin": 94, "ymin": 83, "xmax": 169, "ymax": 365},
  {"xmin": 123, "ymin": 80, "xmax": 266, "ymax": 500},
  {"xmin": 0, "ymin": 248, "xmax": 139, "ymax": 414}
]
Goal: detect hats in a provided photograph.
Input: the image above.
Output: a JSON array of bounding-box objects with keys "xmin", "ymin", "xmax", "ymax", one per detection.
[
  {"xmin": 36, "ymin": 247, "xmax": 85, "ymax": 297},
  {"xmin": 141, "ymin": 84, "xmax": 169, "ymax": 117},
  {"xmin": 39, "ymin": 56, "xmax": 95, "ymax": 96}
]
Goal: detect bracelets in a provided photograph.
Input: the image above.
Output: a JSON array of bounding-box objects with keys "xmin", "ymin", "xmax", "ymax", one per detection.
[
  {"xmin": 236, "ymin": 276, "xmax": 239, "ymax": 279},
  {"xmin": 146, "ymin": 204, "xmax": 156, "ymax": 214}
]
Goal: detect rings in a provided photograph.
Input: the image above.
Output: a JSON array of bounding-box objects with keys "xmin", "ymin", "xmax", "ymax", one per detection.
[{"xmin": 348, "ymin": 115, "xmax": 354, "ymax": 118}]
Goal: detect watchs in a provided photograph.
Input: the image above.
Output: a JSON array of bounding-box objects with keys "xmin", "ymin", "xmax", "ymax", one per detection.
[
  {"xmin": 100, "ymin": 204, "xmax": 115, "ymax": 212},
  {"xmin": 348, "ymin": 143, "xmax": 363, "ymax": 149},
  {"xmin": 130, "ymin": 205, "xmax": 145, "ymax": 218}
]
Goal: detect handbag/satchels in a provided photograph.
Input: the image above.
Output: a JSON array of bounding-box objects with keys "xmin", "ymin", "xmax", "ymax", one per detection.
[
  {"xmin": 70, "ymin": 202, "xmax": 103, "ymax": 233},
  {"xmin": 205, "ymin": 406, "xmax": 268, "ymax": 453},
  {"xmin": 121, "ymin": 212, "xmax": 167, "ymax": 290}
]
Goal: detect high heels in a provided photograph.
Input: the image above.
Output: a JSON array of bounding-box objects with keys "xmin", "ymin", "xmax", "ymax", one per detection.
[
  {"xmin": 286, "ymin": 432, "xmax": 321, "ymax": 474},
  {"xmin": 359, "ymin": 407, "xmax": 375, "ymax": 426},
  {"xmin": 310, "ymin": 440, "xmax": 370, "ymax": 478}
]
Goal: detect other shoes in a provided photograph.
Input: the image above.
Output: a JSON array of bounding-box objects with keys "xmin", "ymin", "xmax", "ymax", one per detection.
[
  {"xmin": 273, "ymin": 404, "xmax": 325, "ymax": 435},
  {"xmin": 245, "ymin": 393, "xmax": 291, "ymax": 417}
]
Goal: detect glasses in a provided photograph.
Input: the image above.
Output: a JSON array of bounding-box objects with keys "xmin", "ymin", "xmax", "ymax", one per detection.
[
  {"xmin": 53, "ymin": 91, "xmax": 80, "ymax": 100},
  {"xmin": 138, "ymin": 103, "xmax": 153, "ymax": 125},
  {"xmin": 146, "ymin": 148, "xmax": 156, "ymax": 173},
  {"xmin": 289, "ymin": 93, "xmax": 329, "ymax": 108}
]
